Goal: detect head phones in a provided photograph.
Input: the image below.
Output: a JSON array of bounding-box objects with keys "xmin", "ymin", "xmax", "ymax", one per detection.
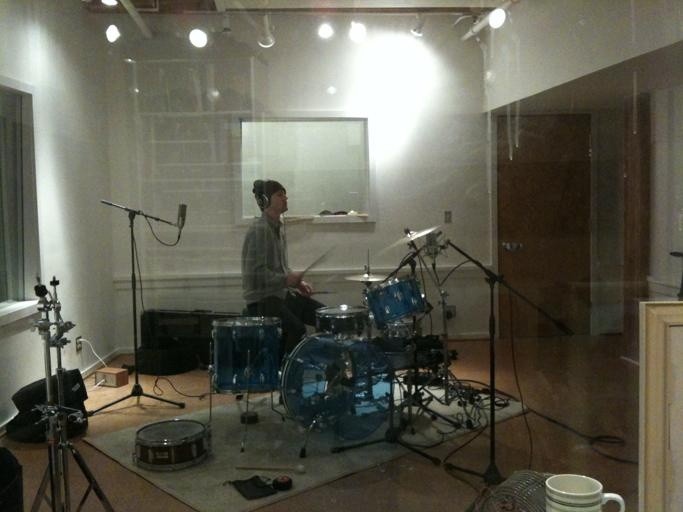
[{"xmin": 256, "ymin": 181, "xmax": 270, "ymax": 210}]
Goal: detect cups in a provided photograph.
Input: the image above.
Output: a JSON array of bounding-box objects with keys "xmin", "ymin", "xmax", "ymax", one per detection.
[{"xmin": 544, "ymin": 474, "xmax": 626, "ymax": 511}]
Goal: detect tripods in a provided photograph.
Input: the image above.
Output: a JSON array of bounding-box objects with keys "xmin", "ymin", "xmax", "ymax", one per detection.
[
  {"xmin": 87, "ymin": 198, "xmax": 176, "ymax": 416},
  {"xmin": 446, "ymin": 238, "xmax": 573, "ymax": 502},
  {"xmin": 332, "ymin": 292, "xmax": 477, "ymax": 469},
  {"xmin": 27, "ymin": 275, "xmax": 117, "ymax": 512}
]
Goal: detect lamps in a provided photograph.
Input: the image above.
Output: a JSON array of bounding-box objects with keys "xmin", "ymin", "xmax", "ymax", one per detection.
[
  {"xmin": 410, "ymin": 12, "xmax": 429, "ymax": 39},
  {"xmin": 253, "ymin": 12, "xmax": 274, "ymax": 50},
  {"xmin": 460, "ymin": 7, "xmax": 508, "ymax": 45}
]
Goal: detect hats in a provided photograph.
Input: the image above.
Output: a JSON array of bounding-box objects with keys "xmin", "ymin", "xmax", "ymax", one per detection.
[{"xmin": 252, "ymin": 179, "xmax": 286, "ymax": 210}]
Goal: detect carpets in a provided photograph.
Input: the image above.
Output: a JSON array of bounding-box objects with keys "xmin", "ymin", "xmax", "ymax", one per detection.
[{"xmin": 81, "ymin": 368, "xmax": 529, "ymax": 511}]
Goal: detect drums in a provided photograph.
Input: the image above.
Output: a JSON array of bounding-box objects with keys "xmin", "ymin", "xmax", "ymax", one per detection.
[
  {"xmin": 212, "ymin": 315, "xmax": 283, "ymax": 395},
  {"xmin": 316, "ymin": 304, "xmax": 370, "ymax": 334},
  {"xmin": 281, "ymin": 332, "xmax": 393, "ymax": 445},
  {"xmin": 365, "ymin": 277, "xmax": 433, "ymax": 330},
  {"xmin": 132, "ymin": 418, "xmax": 210, "ymax": 474}
]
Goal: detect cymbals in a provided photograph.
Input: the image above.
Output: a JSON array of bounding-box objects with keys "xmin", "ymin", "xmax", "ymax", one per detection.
[
  {"xmin": 345, "ymin": 275, "xmax": 387, "ymax": 283},
  {"xmin": 382, "ymin": 225, "xmax": 439, "ymax": 253}
]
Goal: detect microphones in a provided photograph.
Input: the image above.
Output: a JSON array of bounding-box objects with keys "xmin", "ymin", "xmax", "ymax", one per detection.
[{"xmin": 176, "ymin": 202, "xmax": 187, "ymax": 230}]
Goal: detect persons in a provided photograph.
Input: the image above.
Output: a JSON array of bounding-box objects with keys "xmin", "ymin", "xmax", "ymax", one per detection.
[{"xmin": 242, "ymin": 180, "xmax": 331, "ymax": 350}]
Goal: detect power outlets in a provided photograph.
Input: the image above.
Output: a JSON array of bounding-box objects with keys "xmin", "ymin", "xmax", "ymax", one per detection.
[
  {"xmin": 445, "ymin": 304, "xmax": 455, "ymax": 321},
  {"xmin": 75, "ymin": 335, "xmax": 82, "ymax": 353}
]
